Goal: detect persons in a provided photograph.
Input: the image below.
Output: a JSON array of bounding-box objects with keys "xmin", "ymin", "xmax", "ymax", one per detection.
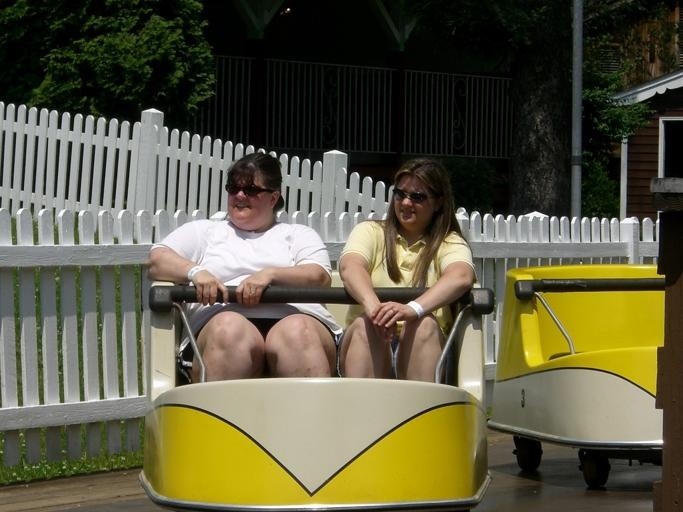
[
  {"xmin": 148, "ymin": 151, "xmax": 346, "ymax": 386},
  {"xmin": 335, "ymin": 156, "xmax": 479, "ymax": 382}
]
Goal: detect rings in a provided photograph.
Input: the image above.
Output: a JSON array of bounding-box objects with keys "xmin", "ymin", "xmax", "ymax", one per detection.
[{"xmin": 390, "ymin": 308, "xmax": 396, "ymax": 314}]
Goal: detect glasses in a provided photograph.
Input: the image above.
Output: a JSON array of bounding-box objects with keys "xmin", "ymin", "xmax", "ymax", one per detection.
[
  {"xmin": 224, "ymin": 183, "xmax": 274, "ymax": 196},
  {"xmin": 392, "ymin": 189, "xmax": 434, "ymax": 203}
]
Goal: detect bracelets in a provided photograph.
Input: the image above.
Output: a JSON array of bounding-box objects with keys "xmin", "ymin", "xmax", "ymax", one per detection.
[
  {"xmin": 187, "ymin": 265, "xmax": 207, "ymax": 282},
  {"xmin": 407, "ymin": 301, "xmax": 424, "ymax": 320}
]
